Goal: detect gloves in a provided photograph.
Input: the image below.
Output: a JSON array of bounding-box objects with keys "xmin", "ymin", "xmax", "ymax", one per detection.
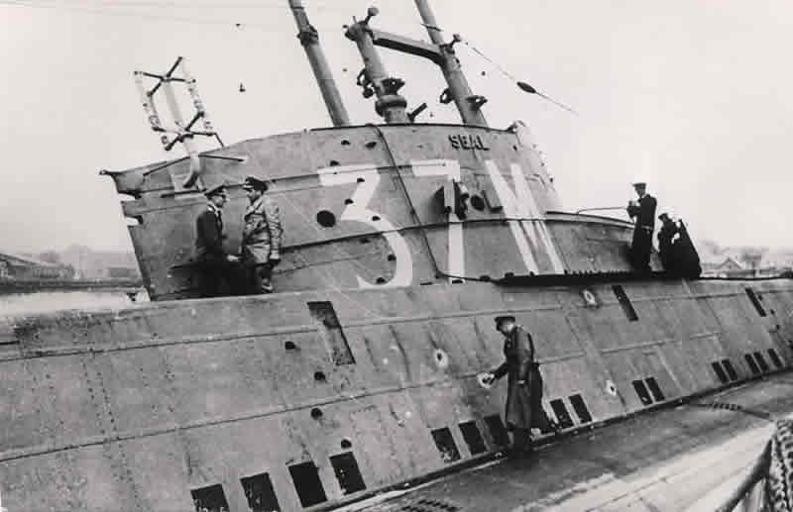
[{"xmin": 268, "ymin": 250, "xmax": 281, "ymax": 266}]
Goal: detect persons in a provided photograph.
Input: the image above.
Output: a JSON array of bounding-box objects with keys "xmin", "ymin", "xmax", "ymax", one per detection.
[
  {"xmin": 656, "ymin": 214, "xmax": 678, "ymax": 272},
  {"xmin": 482, "ymin": 311, "xmax": 544, "ymax": 457},
  {"xmin": 625, "ymin": 183, "xmax": 658, "ymax": 271},
  {"xmin": 240, "ymin": 176, "xmax": 283, "ymax": 295},
  {"xmin": 195, "ymin": 184, "xmax": 229, "ymax": 299}
]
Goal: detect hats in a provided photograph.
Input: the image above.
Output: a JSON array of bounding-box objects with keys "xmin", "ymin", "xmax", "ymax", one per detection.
[
  {"xmin": 204, "ymin": 183, "xmax": 224, "ymax": 200},
  {"xmin": 632, "ymin": 182, "xmax": 646, "ymax": 189},
  {"xmin": 242, "ymin": 176, "xmax": 268, "ymax": 194},
  {"xmin": 494, "ymin": 315, "xmax": 515, "ymax": 329}
]
